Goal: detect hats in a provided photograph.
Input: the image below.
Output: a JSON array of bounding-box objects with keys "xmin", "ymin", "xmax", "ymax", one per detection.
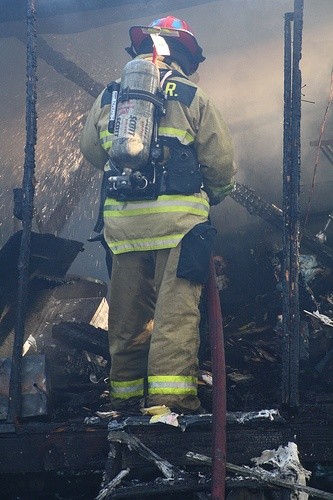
[{"xmin": 128, "ymin": 16, "xmax": 204, "ymax": 62}]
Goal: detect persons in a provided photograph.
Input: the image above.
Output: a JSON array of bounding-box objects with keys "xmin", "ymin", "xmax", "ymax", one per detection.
[{"xmin": 80, "ymin": 16, "xmax": 236, "ymax": 416}]
